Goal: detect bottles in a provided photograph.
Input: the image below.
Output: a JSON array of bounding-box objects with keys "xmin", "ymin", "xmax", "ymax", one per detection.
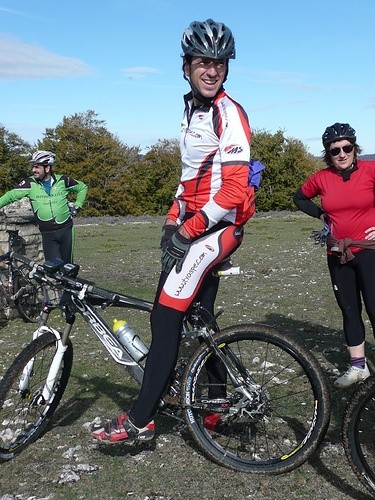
[{"xmin": 112, "ymin": 318, "xmax": 149, "ymax": 365}]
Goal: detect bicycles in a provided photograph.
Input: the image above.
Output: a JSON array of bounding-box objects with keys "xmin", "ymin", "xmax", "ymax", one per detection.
[
  {"xmin": 0, "ymin": 251, "xmax": 332, "ymax": 476},
  {"xmin": 0, "ymin": 229, "xmax": 49, "ymax": 328},
  {"xmin": 341, "ymin": 374, "xmax": 375, "ymax": 497}
]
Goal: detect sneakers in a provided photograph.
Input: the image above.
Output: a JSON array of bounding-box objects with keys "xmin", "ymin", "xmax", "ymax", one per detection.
[
  {"xmin": 333, "ymin": 358, "xmax": 370, "ymax": 388},
  {"xmin": 203, "ymin": 403, "xmax": 231, "ymax": 429},
  {"xmin": 88, "ymin": 410, "xmax": 155, "ymax": 444}
]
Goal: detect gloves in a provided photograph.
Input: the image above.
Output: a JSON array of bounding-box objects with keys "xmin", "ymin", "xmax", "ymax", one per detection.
[
  {"xmin": 160, "ymin": 225, "xmax": 178, "ymax": 250},
  {"xmin": 70, "ymin": 206, "xmax": 81, "ymax": 218},
  {"xmin": 160, "ymin": 231, "xmax": 196, "ymax": 274},
  {"xmin": 310, "ymin": 217, "xmax": 330, "ymax": 247}
]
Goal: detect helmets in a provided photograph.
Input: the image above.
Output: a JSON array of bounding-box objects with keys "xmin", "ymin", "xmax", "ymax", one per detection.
[
  {"xmin": 180, "ymin": 18, "xmax": 236, "ymax": 59},
  {"xmin": 322, "ymin": 122, "xmax": 356, "ymax": 145},
  {"xmin": 29, "ymin": 151, "xmax": 57, "ymax": 166}
]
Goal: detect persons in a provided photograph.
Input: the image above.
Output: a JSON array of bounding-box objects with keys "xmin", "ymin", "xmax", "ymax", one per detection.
[
  {"xmin": 89, "ymin": 18, "xmax": 252, "ymax": 446},
  {"xmin": 293, "ymin": 122, "xmax": 375, "ymax": 389},
  {"xmin": 0, "ymin": 149, "xmax": 88, "ymax": 264}
]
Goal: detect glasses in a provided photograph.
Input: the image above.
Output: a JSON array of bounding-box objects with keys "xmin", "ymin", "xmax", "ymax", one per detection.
[
  {"xmin": 327, "ymin": 145, "xmax": 354, "ymax": 156},
  {"xmin": 32, "ymin": 165, "xmax": 43, "ymax": 168}
]
